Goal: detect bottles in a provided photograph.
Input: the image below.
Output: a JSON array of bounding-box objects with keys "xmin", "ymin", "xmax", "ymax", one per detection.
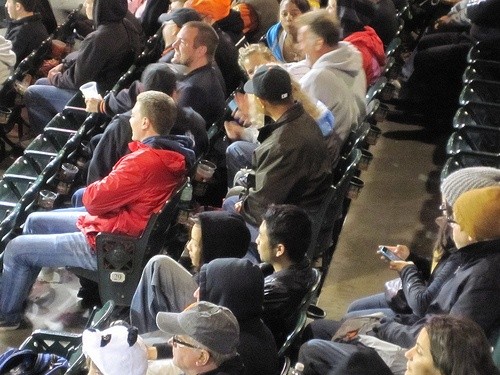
[
  {"xmin": 178, "ymin": 177, "xmax": 192, "ymax": 222},
  {"xmin": 287, "ymin": 362, "xmax": 305, "ymax": 375}
]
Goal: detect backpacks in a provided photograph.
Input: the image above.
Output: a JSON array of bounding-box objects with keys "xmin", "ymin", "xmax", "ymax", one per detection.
[{"xmin": 0, "ymin": 348, "xmax": 69, "ymax": 375}]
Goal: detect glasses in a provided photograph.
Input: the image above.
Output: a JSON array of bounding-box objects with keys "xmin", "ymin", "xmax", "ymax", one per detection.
[
  {"xmin": 438, "ymin": 202, "xmax": 451, "ymax": 214},
  {"xmin": 173, "ymin": 334, "xmax": 197, "ymax": 349},
  {"xmin": 447, "ymin": 216, "xmax": 456, "ymax": 229}
]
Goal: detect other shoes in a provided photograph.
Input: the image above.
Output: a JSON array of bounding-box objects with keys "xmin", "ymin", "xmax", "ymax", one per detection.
[{"xmin": 0, "ymin": 322, "xmax": 20, "ymax": 331}]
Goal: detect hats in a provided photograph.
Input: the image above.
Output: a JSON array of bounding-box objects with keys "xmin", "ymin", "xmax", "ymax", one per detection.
[
  {"xmin": 141, "ymin": 63, "xmax": 177, "ymax": 97},
  {"xmin": 452, "ymin": 187, "xmax": 500, "ymax": 240},
  {"xmin": 244, "ymin": 65, "xmax": 291, "ymax": 101},
  {"xmin": 441, "ymin": 167, "xmax": 500, "ymax": 207},
  {"xmin": 158, "ymin": 8, "xmax": 201, "ymax": 28},
  {"xmin": 156, "ymin": 301, "xmax": 240, "ymax": 353}
]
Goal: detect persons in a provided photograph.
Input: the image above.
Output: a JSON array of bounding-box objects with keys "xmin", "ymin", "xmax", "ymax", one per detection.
[
  {"xmin": 295, "ymin": 167, "xmax": 500, "ymax": 375},
  {"xmin": 395, "ymin": 0, "xmax": 500, "ymax": 109},
  {"xmin": 0, "ymin": 91, "xmax": 317, "ymax": 375},
  {"xmin": 163, "ymin": 0, "xmax": 399, "ymax": 244},
  {"xmin": 0, "ymin": 0, "xmax": 171, "ymax": 142}
]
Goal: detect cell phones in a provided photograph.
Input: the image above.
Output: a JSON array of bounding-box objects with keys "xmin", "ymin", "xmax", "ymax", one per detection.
[{"xmin": 379, "ymin": 245, "xmax": 401, "ymax": 261}]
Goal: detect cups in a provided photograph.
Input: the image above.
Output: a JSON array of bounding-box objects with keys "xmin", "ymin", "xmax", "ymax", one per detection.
[
  {"xmin": 56, "ymin": 163, "xmax": 79, "ymax": 196},
  {"xmin": 49, "ymin": 40, "xmax": 66, "ymax": 59},
  {"xmin": 79, "ymin": 81, "xmax": 98, "ymax": 102},
  {"xmin": 0, "ymin": 105, "xmax": 12, "ymax": 123},
  {"xmin": 193, "ymin": 159, "xmax": 217, "ymax": 197},
  {"xmin": 37, "ymin": 190, "xmax": 57, "ymax": 211}
]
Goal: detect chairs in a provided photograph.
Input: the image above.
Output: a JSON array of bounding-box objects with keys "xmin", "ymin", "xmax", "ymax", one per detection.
[{"xmin": 0, "ymin": 0, "xmax": 500, "ymax": 375}]
